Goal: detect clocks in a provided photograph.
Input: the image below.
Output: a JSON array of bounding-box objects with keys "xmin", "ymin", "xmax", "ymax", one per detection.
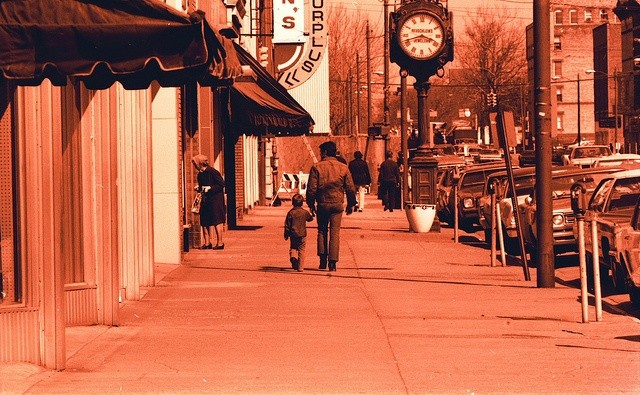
[{"xmin": 395, "ymin": 10, "xmax": 447, "ymax": 61}]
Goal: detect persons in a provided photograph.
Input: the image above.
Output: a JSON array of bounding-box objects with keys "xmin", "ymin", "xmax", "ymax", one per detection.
[
  {"xmin": 306, "ymin": 141, "xmax": 356, "ymax": 272},
  {"xmin": 349, "ymin": 151, "xmax": 371, "ymax": 212},
  {"xmin": 378, "ymin": 151, "xmax": 400, "ymax": 212},
  {"xmin": 191, "ymin": 154, "xmax": 227, "ymax": 250},
  {"xmin": 283, "ymin": 194, "xmax": 313, "ymax": 272}
]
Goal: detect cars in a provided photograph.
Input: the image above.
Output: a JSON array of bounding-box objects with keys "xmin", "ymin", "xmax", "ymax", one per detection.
[
  {"xmin": 457, "ymin": 162, "xmax": 522, "ymax": 230},
  {"xmin": 518, "ymin": 170, "xmax": 597, "ymax": 263},
  {"xmin": 478, "ymin": 165, "xmax": 579, "ymax": 251},
  {"xmin": 592, "ymin": 155, "xmax": 640, "ymax": 171},
  {"xmin": 560, "ymin": 145, "xmax": 613, "ymax": 171},
  {"xmin": 410, "ymin": 142, "xmax": 462, "ymax": 214},
  {"xmin": 608, "ymin": 192, "xmax": 640, "ymax": 303}
]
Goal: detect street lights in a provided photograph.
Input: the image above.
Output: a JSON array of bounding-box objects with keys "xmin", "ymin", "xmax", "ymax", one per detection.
[
  {"xmin": 585, "ymin": 68, "xmax": 619, "ymax": 143},
  {"xmin": 363, "ymin": 21, "xmax": 383, "ymax": 131},
  {"xmin": 553, "ymin": 73, "xmax": 580, "ymax": 139},
  {"xmin": 355, "ymin": 50, "xmax": 374, "ymax": 133},
  {"xmin": 346, "ymin": 73, "xmax": 362, "ymax": 135},
  {"xmin": 349, "ymin": 68, "xmax": 367, "ymax": 133}
]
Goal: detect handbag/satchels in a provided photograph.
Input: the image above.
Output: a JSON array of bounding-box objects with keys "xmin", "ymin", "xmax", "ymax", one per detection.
[{"xmin": 191, "ymin": 188, "xmax": 204, "ymax": 215}]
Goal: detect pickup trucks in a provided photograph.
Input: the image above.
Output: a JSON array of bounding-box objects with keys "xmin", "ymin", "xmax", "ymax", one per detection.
[{"xmin": 572, "ymin": 169, "xmax": 640, "ymax": 292}]
